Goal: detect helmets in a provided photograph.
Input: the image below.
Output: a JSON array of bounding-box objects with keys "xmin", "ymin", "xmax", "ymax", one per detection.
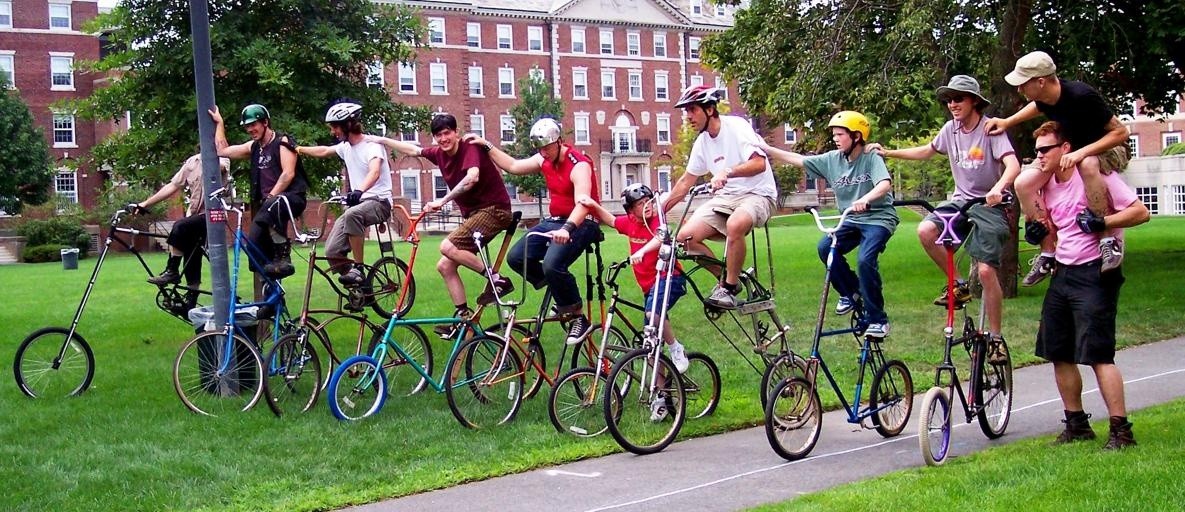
[
  {"xmin": 240, "ymin": 104, "xmax": 271, "ymax": 125},
  {"xmin": 828, "ymin": 111, "xmax": 870, "ymax": 145},
  {"xmin": 529, "ymin": 118, "xmax": 561, "ymax": 149},
  {"xmin": 674, "ymin": 85, "xmax": 721, "ymax": 108},
  {"xmin": 620, "ymin": 184, "xmax": 653, "ymax": 212},
  {"xmin": 323, "ymin": 98, "xmax": 363, "ymax": 123}
]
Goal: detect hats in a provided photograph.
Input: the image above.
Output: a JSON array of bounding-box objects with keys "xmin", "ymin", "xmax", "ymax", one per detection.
[
  {"xmin": 1005, "ymin": 51, "xmax": 1056, "ymax": 86},
  {"xmin": 936, "ymin": 75, "xmax": 991, "ymax": 108}
]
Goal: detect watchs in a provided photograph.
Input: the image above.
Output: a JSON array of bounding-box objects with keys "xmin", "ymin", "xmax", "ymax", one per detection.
[{"xmin": 724, "ymin": 167, "xmax": 733, "ymax": 178}]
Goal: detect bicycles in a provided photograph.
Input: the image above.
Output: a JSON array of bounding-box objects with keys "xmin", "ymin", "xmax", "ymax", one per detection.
[
  {"xmin": 445, "ymin": 230, "xmax": 633, "ymax": 427},
  {"xmin": 602, "ymin": 184, "xmax": 815, "ymax": 456},
  {"xmin": 329, "ymin": 205, "xmax": 545, "ymax": 417},
  {"xmin": 549, "ymin": 259, "xmax": 721, "ymax": 440},
  {"xmin": 765, "ymin": 204, "xmax": 914, "ymax": 464},
  {"xmin": 891, "ymin": 196, "xmax": 1014, "ymax": 468},
  {"xmin": 258, "ymin": 194, "xmax": 432, "ymax": 418},
  {"xmin": 175, "ymin": 182, "xmax": 334, "ymax": 416},
  {"xmin": 14, "ymin": 203, "xmax": 286, "ymax": 398}
]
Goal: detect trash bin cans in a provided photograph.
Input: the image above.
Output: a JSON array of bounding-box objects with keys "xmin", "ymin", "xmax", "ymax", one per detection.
[
  {"xmin": 187, "ymin": 306, "xmax": 263, "ymax": 393},
  {"xmin": 60, "ymin": 246, "xmax": 80, "ymax": 271}
]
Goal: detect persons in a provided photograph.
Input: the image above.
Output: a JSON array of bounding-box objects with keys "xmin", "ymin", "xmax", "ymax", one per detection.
[
  {"xmin": 363, "ymin": 114, "xmax": 515, "ymax": 338},
  {"xmin": 653, "ymin": 84, "xmax": 780, "ymax": 310},
  {"xmin": 207, "ymin": 104, "xmax": 308, "ymax": 320},
  {"xmin": 862, "ymin": 75, "xmax": 1020, "ymax": 365},
  {"xmin": 1024, "ymin": 121, "xmax": 1151, "ymax": 448},
  {"xmin": 983, "ymin": 51, "xmax": 1131, "ymax": 287},
  {"xmin": 293, "ymin": 102, "xmax": 394, "ymax": 310},
  {"xmin": 462, "ymin": 116, "xmax": 602, "ymax": 346},
  {"xmin": 129, "ymin": 140, "xmax": 237, "ymax": 320},
  {"xmin": 576, "ymin": 182, "xmax": 691, "ymax": 424},
  {"xmin": 751, "ymin": 111, "xmax": 900, "ymax": 337}
]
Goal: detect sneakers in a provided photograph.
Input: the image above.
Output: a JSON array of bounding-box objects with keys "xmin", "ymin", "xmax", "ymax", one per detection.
[
  {"xmin": 1056, "ymin": 415, "xmax": 1095, "ymax": 444},
  {"xmin": 1103, "ymin": 417, "xmax": 1135, "ymax": 448}
]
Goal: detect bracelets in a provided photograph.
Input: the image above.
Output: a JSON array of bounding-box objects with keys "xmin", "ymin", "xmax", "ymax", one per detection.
[
  {"xmin": 297, "ymin": 146, "xmax": 302, "ymax": 155},
  {"xmin": 561, "ymin": 220, "xmax": 577, "ymax": 236},
  {"xmin": 483, "ymin": 142, "xmax": 494, "ymax": 151}
]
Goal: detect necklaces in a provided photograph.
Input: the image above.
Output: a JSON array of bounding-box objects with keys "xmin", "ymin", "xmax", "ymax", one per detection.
[{"xmin": 266, "ymin": 130, "xmax": 274, "ymax": 144}]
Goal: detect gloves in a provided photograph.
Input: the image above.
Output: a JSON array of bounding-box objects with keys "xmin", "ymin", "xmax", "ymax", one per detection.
[
  {"xmin": 1076, "ymin": 209, "xmax": 1105, "ymax": 233},
  {"xmin": 346, "ymin": 190, "xmax": 362, "ymax": 205},
  {"xmin": 1024, "ymin": 220, "xmax": 1047, "ymax": 244},
  {"xmin": 289, "ymin": 136, "xmax": 298, "ymax": 149}
]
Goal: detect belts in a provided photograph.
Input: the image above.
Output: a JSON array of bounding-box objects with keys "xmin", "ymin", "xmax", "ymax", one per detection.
[{"xmin": 551, "ymin": 214, "xmax": 600, "ymax": 224}]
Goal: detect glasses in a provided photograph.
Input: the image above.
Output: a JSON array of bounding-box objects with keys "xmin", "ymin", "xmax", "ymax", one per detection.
[
  {"xmin": 1033, "ymin": 144, "xmax": 1060, "ymax": 154},
  {"xmin": 947, "ymin": 95, "xmax": 969, "ymax": 103}
]
